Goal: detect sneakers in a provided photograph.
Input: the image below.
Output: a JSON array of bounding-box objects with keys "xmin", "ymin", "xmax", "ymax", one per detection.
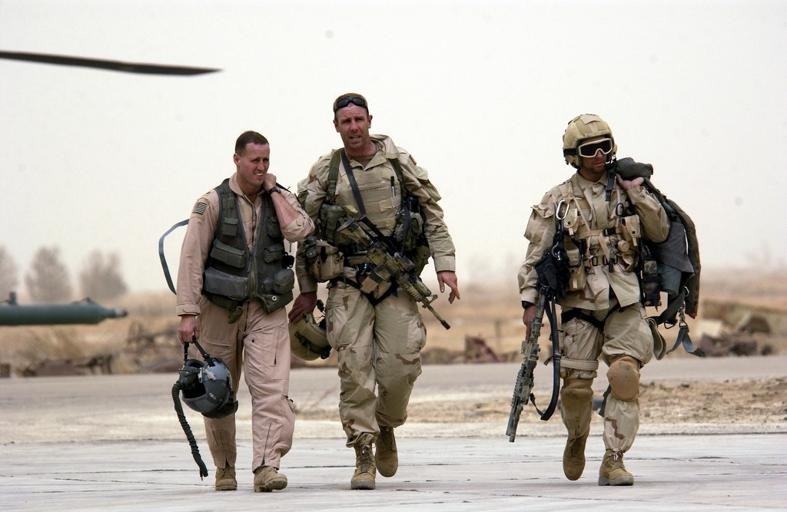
[
  {"xmin": 214, "ymin": 465, "xmax": 239, "ymax": 492},
  {"xmin": 559, "ymin": 422, "xmax": 591, "ymax": 482},
  {"xmin": 374, "ymin": 428, "xmax": 399, "ymax": 478},
  {"xmin": 347, "ymin": 442, "xmax": 377, "ymax": 492},
  {"xmin": 594, "ymin": 449, "xmax": 637, "ymax": 488},
  {"xmin": 253, "ymin": 466, "xmax": 288, "ymax": 493}
]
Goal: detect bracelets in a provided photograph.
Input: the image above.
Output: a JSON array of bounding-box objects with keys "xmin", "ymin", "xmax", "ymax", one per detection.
[{"xmin": 268, "ymin": 186, "xmax": 281, "ymax": 195}]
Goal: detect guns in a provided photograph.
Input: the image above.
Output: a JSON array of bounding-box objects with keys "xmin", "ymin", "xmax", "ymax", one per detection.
[
  {"xmin": 297, "ymin": 189, "xmax": 450, "ymax": 330},
  {"xmin": 504, "ymin": 278, "xmax": 548, "ymax": 443}
]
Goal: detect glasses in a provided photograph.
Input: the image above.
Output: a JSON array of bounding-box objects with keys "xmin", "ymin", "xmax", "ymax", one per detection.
[{"xmin": 578, "ymin": 136, "xmax": 613, "ymax": 159}]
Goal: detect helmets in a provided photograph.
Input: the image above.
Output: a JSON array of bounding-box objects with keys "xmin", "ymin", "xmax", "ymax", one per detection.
[
  {"xmin": 561, "ymin": 111, "xmax": 618, "ymax": 169},
  {"xmin": 176, "ymin": 354, "xmax": 240, "ymax": 420},
  {"xmin": 287, "ymin": 310, "xmax": 335, "ymax": 361}
]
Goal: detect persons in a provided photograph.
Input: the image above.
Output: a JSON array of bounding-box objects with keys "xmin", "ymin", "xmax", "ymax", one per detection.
[
  {"xmin": 174, "ymin": 130, "xmax": 314, "ymax": 493},
  {"xmin": 520, "ymin": 112, "xmax": 670, "ymax": 486},
  {"xmin": 287, "ymin": 90, "xmax": 461, "ymax": 490}
]
